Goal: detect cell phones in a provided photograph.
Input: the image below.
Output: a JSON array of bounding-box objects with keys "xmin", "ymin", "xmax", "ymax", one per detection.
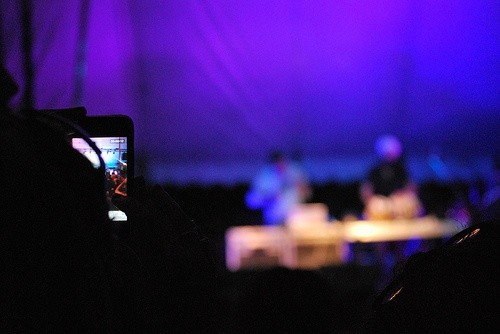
[{"xmin": 67, "ymin": 115, "xmax": 134, "ymax": 237}]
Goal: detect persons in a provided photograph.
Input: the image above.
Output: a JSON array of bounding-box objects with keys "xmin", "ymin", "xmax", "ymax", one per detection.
[
  {"xmin": 247, "ymin": 146, "xmax": 315, "ymax": 225},
  {"xmin": 359, "ymin": 133, "xmax": 420, "ymax": 215},
  {"xmin": 99, "ymin": 151, "xmax": 129, "ymax": 217}
]
